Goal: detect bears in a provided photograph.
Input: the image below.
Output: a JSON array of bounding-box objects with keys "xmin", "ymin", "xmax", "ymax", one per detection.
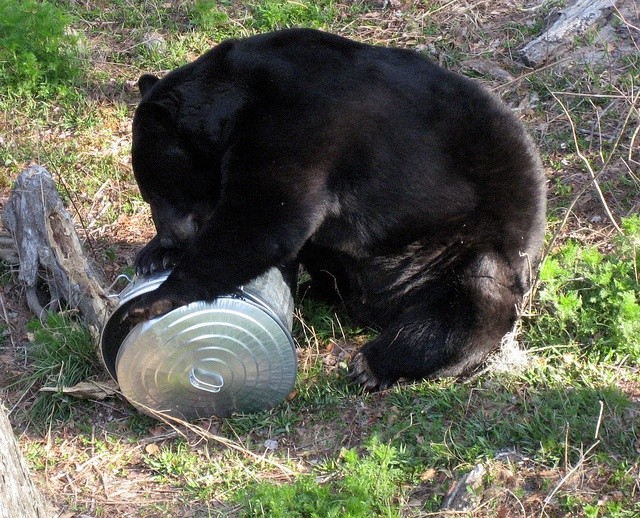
[{"xmin": 131, "ymin": 28, "xmax": 547, "ymax": 394}]
